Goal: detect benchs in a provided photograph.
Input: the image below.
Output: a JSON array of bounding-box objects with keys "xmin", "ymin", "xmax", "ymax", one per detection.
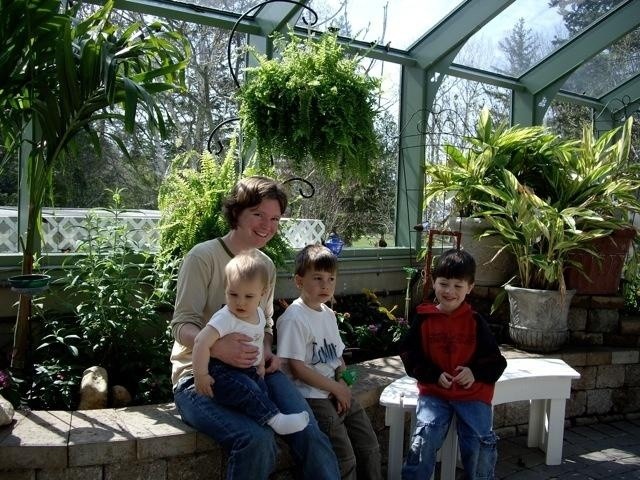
[{"xmin": 378, "ymin": 358, "xmax": 581, "ymax": 480}]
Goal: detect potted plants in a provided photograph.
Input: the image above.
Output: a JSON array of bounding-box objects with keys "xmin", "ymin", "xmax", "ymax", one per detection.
[
  {"xmin": 423, "ymin": 101, "xmax": 572, "ymax": 288},
  {"xmin": 465, "ymin": 165, "xmax": 640, "ymax": 353},
  {"xmin": 538, "ymin": 116, "xmax": 639, "ymax": 297}
]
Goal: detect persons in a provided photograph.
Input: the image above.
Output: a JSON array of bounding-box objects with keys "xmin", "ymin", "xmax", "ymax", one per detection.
[{"xmin": 400, "ymin": 249, "xmax": 507, "ymax": 480}]
